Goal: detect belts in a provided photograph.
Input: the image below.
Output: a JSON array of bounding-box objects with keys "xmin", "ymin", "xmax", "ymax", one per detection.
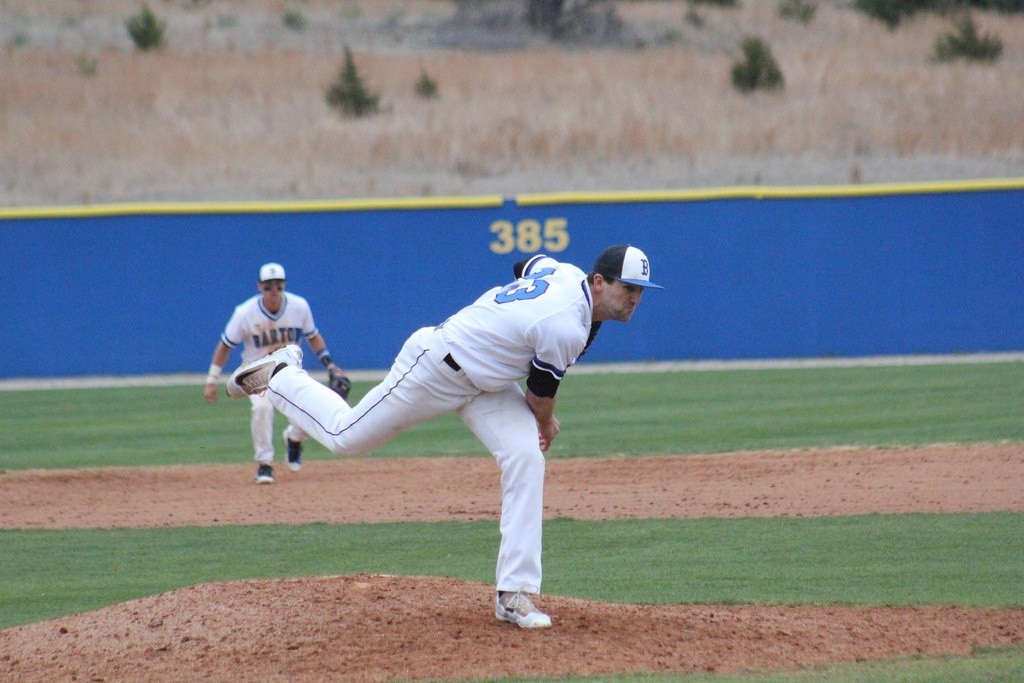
[{"xmin": 443, "ymin": 353, "xmax": 461, "ymax": 372}]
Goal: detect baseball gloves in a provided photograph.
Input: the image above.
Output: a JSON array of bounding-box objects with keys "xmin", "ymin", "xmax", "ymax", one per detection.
[{"xmin": 328, "ymin": 367, "xmax": 351, "ymax": 399}]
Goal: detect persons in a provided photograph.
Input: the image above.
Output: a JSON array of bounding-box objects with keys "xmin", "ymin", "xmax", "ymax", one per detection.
[
  {"xmin": 225, "ymin": 244, "xmax": 665, "ymax": 627},
  {"xmin": 204, "ymin": 263, "xmax": 351, "ymax": 486}
]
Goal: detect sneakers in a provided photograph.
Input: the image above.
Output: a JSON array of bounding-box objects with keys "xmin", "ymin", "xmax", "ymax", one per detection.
[
  {"xmin": 227, "ymin": 344, "xmax": 303, "ymax": 399},
  {"xmin": 496, "ymin": 585, "xmax": 552, "ymax": 627},
  {"xmin": 283, "ymin": 432, "xmax": 303, "ymax": 473},
  {"xmin": 257, "ymin": 462, "xmax": 275, "ymax": 484}
]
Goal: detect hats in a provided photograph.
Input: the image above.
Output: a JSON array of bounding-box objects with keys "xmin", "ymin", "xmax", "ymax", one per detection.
[
  {"xmin": 592, "ymin": 243, "xmax": 664, "ymax": 290},
  {"xmin": 259, "ymin": 263, "xmax": 285, "ymax": 281}
]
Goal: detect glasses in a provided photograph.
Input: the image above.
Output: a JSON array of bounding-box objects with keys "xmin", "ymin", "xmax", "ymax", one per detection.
[{"xmin": 262, "ymin": 285, "xmax": 283, "ymax": 292}]
